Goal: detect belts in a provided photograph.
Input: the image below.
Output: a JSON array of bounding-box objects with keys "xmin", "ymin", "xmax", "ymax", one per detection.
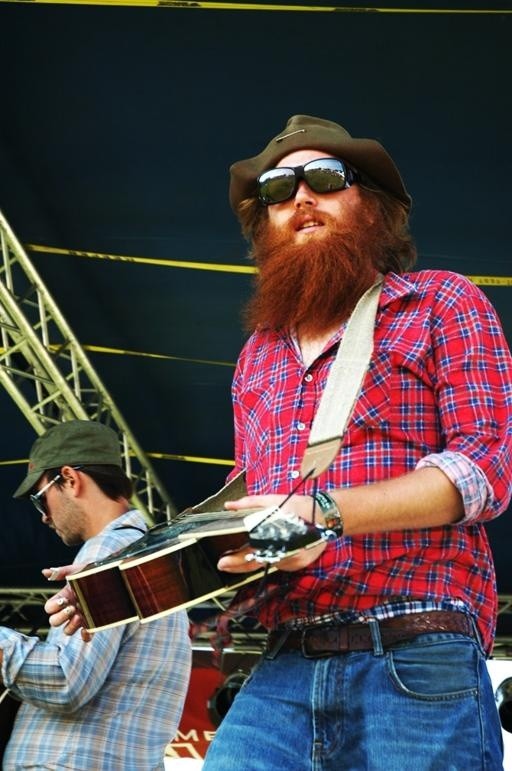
[{"xmin": 266, "ymin": 610, "xmax": 475, "ymax": 659}]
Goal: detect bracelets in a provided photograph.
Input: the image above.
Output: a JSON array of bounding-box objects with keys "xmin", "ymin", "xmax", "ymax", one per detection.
[{"xmin": 314, "ymin": 490, "xmax": 342, "ymax": 543}]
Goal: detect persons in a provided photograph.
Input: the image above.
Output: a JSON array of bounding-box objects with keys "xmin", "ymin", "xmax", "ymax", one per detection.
[
  {"xmin": 43, "ymin": 116, "xmax": 511, "ymax": 770},
  {"xmin": 0, "ymin": 418, "xmax": 191, "ymax": 770}
]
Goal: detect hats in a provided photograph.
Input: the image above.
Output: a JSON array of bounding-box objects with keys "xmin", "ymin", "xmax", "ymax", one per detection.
[
  {"xmin": 228, "ymin": 114, "xmax": 412, "ymax": 219},
  {"xmin": 11, "ymin": 421, "xmax": 122, "ymax": 499}
]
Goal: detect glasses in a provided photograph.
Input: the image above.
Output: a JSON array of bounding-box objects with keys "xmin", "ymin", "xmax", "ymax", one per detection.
[
  {"xmin": 29, "ymin": 466, "xmax": 83, "ymax": 516},
  {"xmin": 255, "ymin": 158, "xmax": 369, "ymax": 206}
]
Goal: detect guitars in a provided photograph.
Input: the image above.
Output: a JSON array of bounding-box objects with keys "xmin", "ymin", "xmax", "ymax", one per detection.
[{"xmin": 67, "ymin": 509, "xmax": 341, "ymax": 634}]
